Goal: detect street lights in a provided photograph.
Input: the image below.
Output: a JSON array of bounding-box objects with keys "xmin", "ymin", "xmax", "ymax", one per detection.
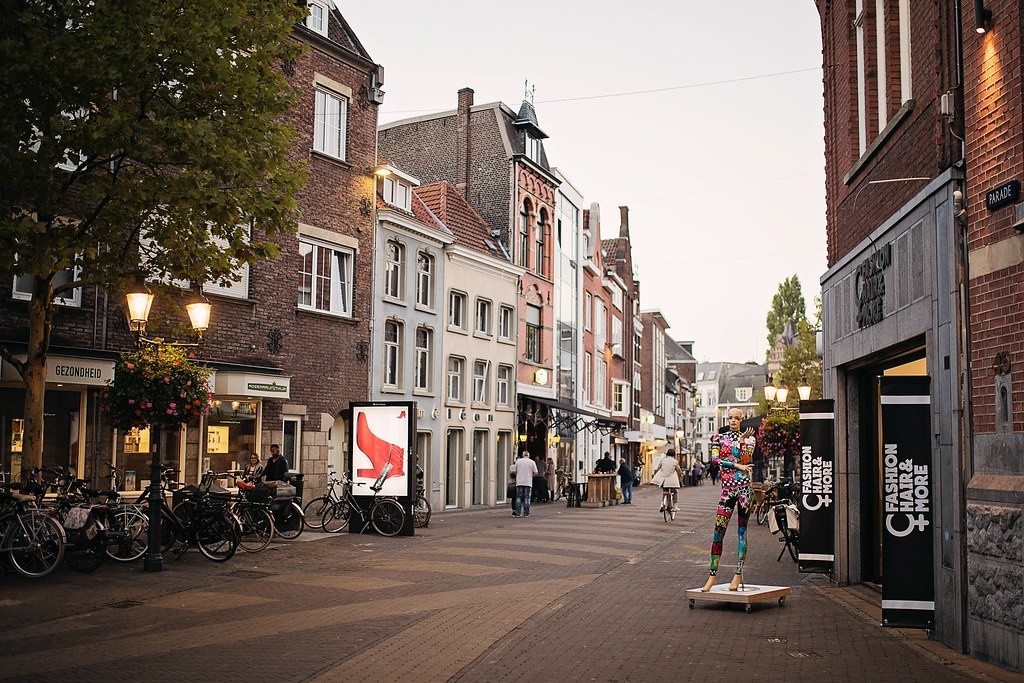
[
  {"xmin": 677, "ymin": 427, "xmax": 684, "ymax": 471},
  {"xmin": 763, "ymin": 372, "xmax": 812, "ymax": 479},
  {"xmin": 124, "ymin": 271, "xmax": 213, "ymax": 574}
]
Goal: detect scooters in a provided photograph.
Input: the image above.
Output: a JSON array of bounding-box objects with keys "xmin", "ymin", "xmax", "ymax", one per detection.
[{"xmin": 633, "ymin": 466, "xmax": 642, "ymax": 486}]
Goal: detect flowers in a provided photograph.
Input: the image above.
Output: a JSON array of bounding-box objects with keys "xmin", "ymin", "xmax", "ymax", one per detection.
[
  {"xmin": 756, "ymin": 413, "xmax": 803, "ymax": 458},
  {"xmin": 94, "ymin": 340, "xmax": 214, "ymax": 435}
]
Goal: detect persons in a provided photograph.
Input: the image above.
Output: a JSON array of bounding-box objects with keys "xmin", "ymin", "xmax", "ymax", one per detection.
[
  {"xmin": 701, "ymin": 408, "xmax": 756, "ymax": 592},
  {"xmin": 692, "ymin": 459, "xmax": 720, "ymax": 486},
  {"xmin": 618, "ymin": 458, "xmax": 634, "ymax": 504},
  {"xmin": 651, "ymin": 449, "xmax": 683, "ymax": 512},
  {"xmin": 533, "ymin": 456, "xmax": 555, "ymax": 502},
  {"xmin": 597, "ymin": 452, "xmax": 615, "ymax": 473},
  {"xmin": 258, "ymin": 444, "xmax": 289, "ymax": 482},
  {"xmin": 508, "ymin": 451, "xmax": 538, "ymax": 517},
  {"xmin": 243, "ymin": 452, "xmax": 263, "ymax": 484}
]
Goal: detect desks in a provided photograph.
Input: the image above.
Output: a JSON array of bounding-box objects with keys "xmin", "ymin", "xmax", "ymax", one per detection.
[{"xmin": 580, "ymin": 473, "xmax": 621, "ymax": 508}]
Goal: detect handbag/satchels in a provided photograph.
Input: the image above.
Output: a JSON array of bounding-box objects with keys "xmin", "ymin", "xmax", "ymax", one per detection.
[
  {"xmin": 767, "ymin": 506, "xmax": 780, "ymax": 533},
  {"xmin": 63, "ymin": 507, "xmax": 99, "ymax": 544},
  {"xmin": 785, "ymin": 504, "xmax": 800, "ymax": 532}
]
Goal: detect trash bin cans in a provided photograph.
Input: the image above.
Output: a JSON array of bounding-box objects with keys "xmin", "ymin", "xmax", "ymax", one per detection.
[
  {"xmin": 173, "ymin": 484, "xmax": 231, "ymax": 544},
  {"xmin": 275, "ymin": 472, "xmax": 305, "ymax": 533},
  {"xmin": 682, "ymin": 470, "xmax": 690, "ymax": 487}
]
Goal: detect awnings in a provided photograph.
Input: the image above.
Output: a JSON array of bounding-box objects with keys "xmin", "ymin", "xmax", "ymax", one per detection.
[{"xmin": 526, "ymin": 395, "xmax": 626, "ymax": 424}]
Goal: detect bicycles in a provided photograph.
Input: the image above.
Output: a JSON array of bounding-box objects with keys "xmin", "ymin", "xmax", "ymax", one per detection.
[
  {"xmin": 553, "ymin": 472, "xmax": 577, "ymax": 502},
  {"xmin": 321, "ymin": 471, "xmax": 407, "ymax": 538},
  {"xmin": 661, "ymin": 476, "xmax": 683, "ymax": 522},
  {"xmin": 303, "ymin": 470, "xmax": 351, "ymax": 530},
  {"xmin": 1, "ymin": 461, "xmax": 305, "ymax": 580},
  {"xmin": 756, "ymin": 480, "xmax": 801, "ymax": 563},
  {"xmin": 414, "ymin": 488, "xmax": 432, "ymax": 529}
]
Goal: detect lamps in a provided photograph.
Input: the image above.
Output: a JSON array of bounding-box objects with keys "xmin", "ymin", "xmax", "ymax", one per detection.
[
  {"xmin": 549, "ymin": 432, "xmax": 560, "ymax": 448},
  {"xmin": 647, "ymin": 449, "xmax": 654, "ymax": 454},
  {"xmin": 973, "ymin": 0, "xmax": 992, "ymax": 34},
  {"xmin": 515, "ymin": 428, "xmax": 527, "ymax": 445},
  {"xmin": 365, "ymin": 165, "xmax": 392, "ymax": 176}
]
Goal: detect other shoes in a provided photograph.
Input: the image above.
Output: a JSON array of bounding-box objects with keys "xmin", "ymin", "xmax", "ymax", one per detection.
[
  {"xmin": 659, "ymin": 506, "xmax": 666, "ymax": 513},
  {"xmin": 620, "ymin": 501, "xmax": 631, "ymax": 505},
  {"xmin": 512, "ymin": 510, "xmax": 522, "ymax": 517},
  {"xmin": 670, "ymin": 507, "xmax": 680, "ymax": 512},
  {"xmin": 524, "ymin": 515, "xmax": 528, "ymax": 518}
]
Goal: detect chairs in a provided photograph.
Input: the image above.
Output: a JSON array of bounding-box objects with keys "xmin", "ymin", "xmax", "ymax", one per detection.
[
  {"xmin": 694, "ymin": 474, "xmax": 702, "ymax": 486},
  {"xmin": 752, "ymin": 480, "xmax": 781, "ymax": 520}
]
